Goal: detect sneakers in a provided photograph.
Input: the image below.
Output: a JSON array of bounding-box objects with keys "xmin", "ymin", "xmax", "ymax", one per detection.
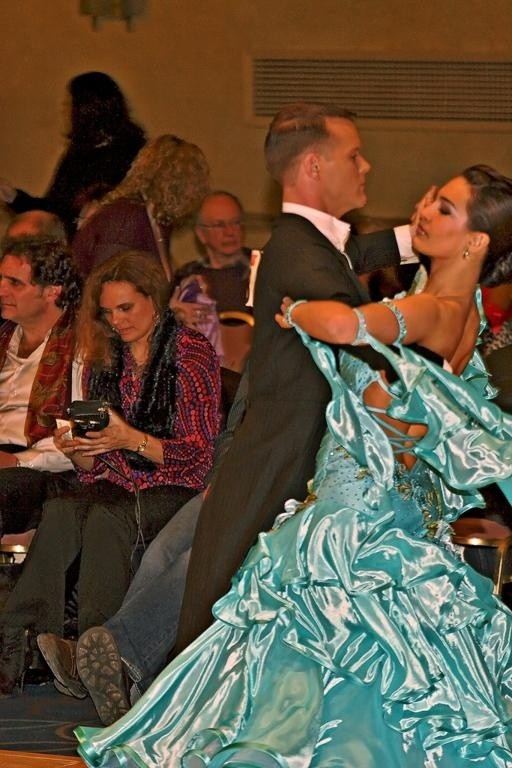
[{"xmin": 1, "ymin": 626, "xmax": 129, "ymax": 728}]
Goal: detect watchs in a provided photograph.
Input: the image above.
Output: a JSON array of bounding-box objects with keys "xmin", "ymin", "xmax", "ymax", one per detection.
[{"xmin": 131, "ymin": 431, "xmax": 151, "ymax": 459}]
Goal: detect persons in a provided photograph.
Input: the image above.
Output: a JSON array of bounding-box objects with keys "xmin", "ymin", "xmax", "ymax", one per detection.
[
  {"xmin": 346, "ymin": 210, "xmax": 427, "ymax": 301},
  {"xmin": 70, "ymin": 133, "xmax": 216, "ymax": 341},
  {"xmin": 169, "ymin": 191, "xmax": 253, "ymax": 326},
  {"xmin": 32, "ymin": 485, "xmax": 206, "ymax": 726},
  {"xmin": 174, "ymin": 99, "xmax": 419, "ymax": 662},
  {"xmin": 3, "ymin": 238, "xmax": 91, "ymax": 539},
  {"xmin": 73, "ymin": 163, "xmax": 511, "ymax": 767},
  {"xmin": 3, "ymin": 210, "xmax": 74, "ymax": 247},
  {"xmin": 4, "ymin": 68, "xmax": 149, "ymax": 231},
  {"xmin": 1, "ymin": 248, "xmax": 228, "ymax": 700}
]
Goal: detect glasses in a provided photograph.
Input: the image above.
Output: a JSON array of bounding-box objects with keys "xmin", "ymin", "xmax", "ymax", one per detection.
[{"xmin": 196, "ymin": 217, "xmax": 248, "ymax": 232}]
